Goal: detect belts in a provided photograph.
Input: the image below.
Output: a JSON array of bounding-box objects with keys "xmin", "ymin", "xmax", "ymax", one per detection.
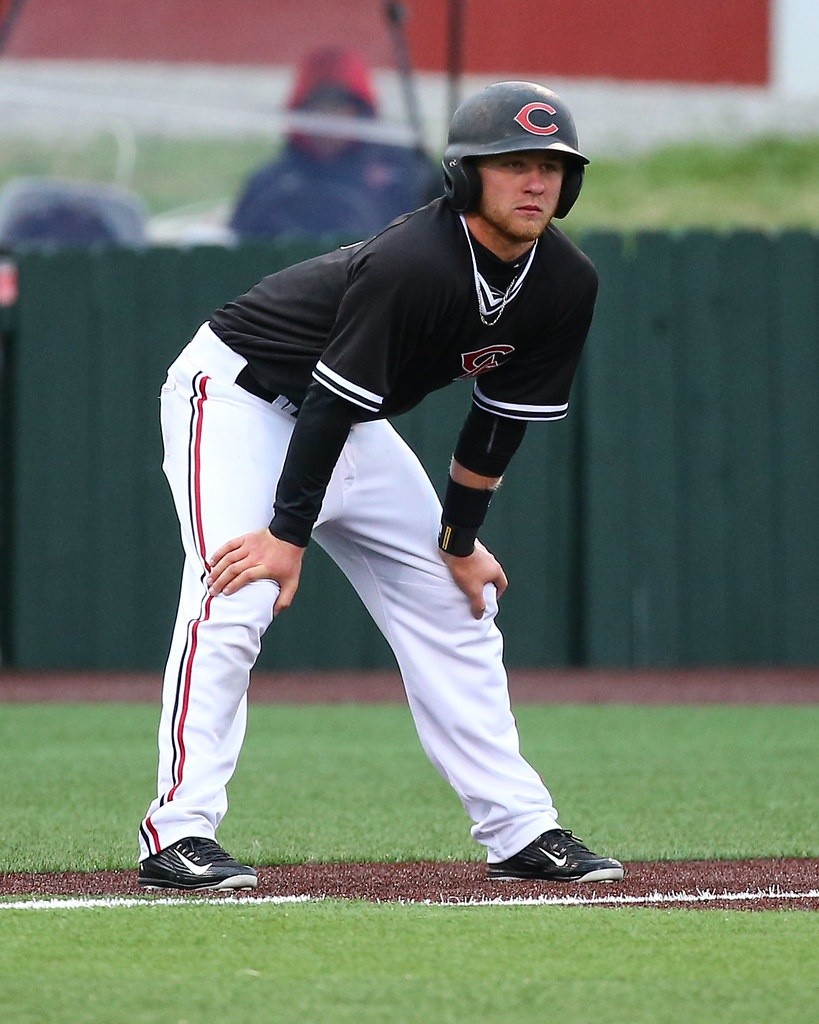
[{"xmin": 236, "ymin": 365, "xmax": 299, "ymax": 418}]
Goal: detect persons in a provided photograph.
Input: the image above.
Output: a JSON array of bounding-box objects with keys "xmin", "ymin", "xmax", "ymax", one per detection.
[
  {"xmin": 224, "ymin": 40, "xmax": 440, "ymax": 243},
  {"xmin": 138, "ymin": 79, "xmax": 626, "ymax": 893}
]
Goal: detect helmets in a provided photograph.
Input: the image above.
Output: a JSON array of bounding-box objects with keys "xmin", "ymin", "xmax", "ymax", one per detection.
[{"xmin": 439, "ymin": 81, "xmax": 590, "ymax": 219}]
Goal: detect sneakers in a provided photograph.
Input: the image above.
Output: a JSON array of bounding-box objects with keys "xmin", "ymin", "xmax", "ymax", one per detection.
[
  {"xmin": 138, "ymin": 835, "xmax": 258, "ymax": 890},
  {"xmin": 483, "ymin": 829, "xmax": 624, "ymax": 884}
]
